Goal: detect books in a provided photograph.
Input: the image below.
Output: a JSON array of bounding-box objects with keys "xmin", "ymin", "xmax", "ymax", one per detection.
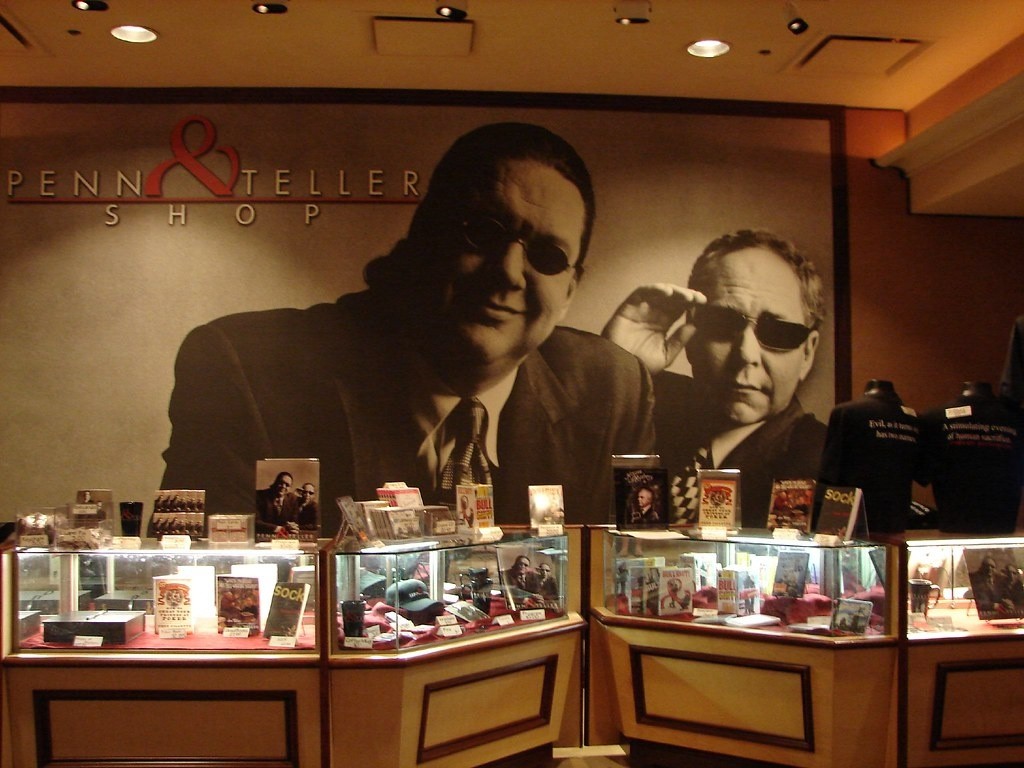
[
  {"xmin": 610, "ymin": 454, "xmax": 890, "ymax": 635},
  {"xmin": 41, "ymin": 455, "xmax": 323, "ymax": 642},
  {"xmin": 964, "ymin": 546, "xmax": 1024, "ymax": 622},
  {"xmin": 335, "ymin": 479, "xmax": 569, "ymax": 618}
]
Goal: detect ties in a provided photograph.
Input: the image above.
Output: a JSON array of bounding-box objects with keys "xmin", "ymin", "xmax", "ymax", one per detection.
[
  {"xmin": 670, "ymin": 441, "xmax": 714, "ymax": 524},
  {"xmin": 277, "ymin": 502, "xmax": 282, "ymax": 514},
  {"xmin": 439, "ymin": 398, "xmax": 493, "ymax": 517}
]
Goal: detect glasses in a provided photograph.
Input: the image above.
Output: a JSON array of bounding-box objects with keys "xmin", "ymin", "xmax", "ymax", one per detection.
[
  {"xmin": 541, "ymin": 569, "xmax": 551, "ymax": 572},
  {"xmin": 1013, "ymin": 572, "xmax": 1017, "ymax": 575},
  {"xmin": 638, "ymin": 496, "xmax": 643, "ymax": 498},
  {"xmin": 987, "ymin": 564, "xmax": 995, "ymax": 568},
  {"xmin": 689, "ymin": 302, "xmax": 816, "ymax": 350},
  {"xmin": 457, "ymin": 209, "xmax": 569, "ymax": 275},
  {"xmin": 519, "ymin": 562, "xmax": 528, "ymax": 567},
  {"xmin": 302, "ymin": 490, "xmax": 314, "ymax": 495},
  {"xmin": 279, "ymin": 481, "xmax": 291, "ymax": 487}
]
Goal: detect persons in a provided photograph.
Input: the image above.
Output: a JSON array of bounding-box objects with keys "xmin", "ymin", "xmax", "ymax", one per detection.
[
  {"xmin": 774, "ymin": 489, "xmax": 810, "ymax": 519},
  {"xmin": 148, "ymin": 494, "xmax": 203, "ymax": 536},
  {"xmin": 148, "ymin": 119, "xmax": 656, "ymax": 528},
  {"xmin": 292, "ymin": 482, "xmax": 320, "ymax": 530},
  {"xmin": 84, "ymin": 490, "xmax": 106, "ymax": 519},
  {"xmin": 599, "ymin": 226, "xmax": 829, "ymax": 528},
  {"xmin": 634, "ymin": 486, "xmax": 661, "ymax": 525},
  {"xmin": 534, "ymin": 561, "xmax": 561, "ymax": 601},
  {"xmin": 254, "ymin": 470, "xmax": 300, "ymax": 534},
  {"xmin": 813, "ymin": 379, "xmax": 930, "ymax": 533},
  {"xmin": 502, "ymin": 555, "xmax": 544, "ymax": 604},
  {"xmin": 923, "ymin": 377, "xmax": 1024, "ymax": 536},
  {"xmin": 973, "ymin": 556, "xmax": 1024, "ymax": 612}
]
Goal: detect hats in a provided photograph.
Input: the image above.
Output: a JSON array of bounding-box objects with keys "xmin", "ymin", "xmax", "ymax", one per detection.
[{"xmin": 386, "ymin": 580, "xmax": 443, "ymax": 611}]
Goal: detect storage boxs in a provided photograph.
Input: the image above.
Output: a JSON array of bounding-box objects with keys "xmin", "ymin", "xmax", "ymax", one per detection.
[{"xmin": 43, "ymin": 611, "xmax": 146, "ymax": 644}]
